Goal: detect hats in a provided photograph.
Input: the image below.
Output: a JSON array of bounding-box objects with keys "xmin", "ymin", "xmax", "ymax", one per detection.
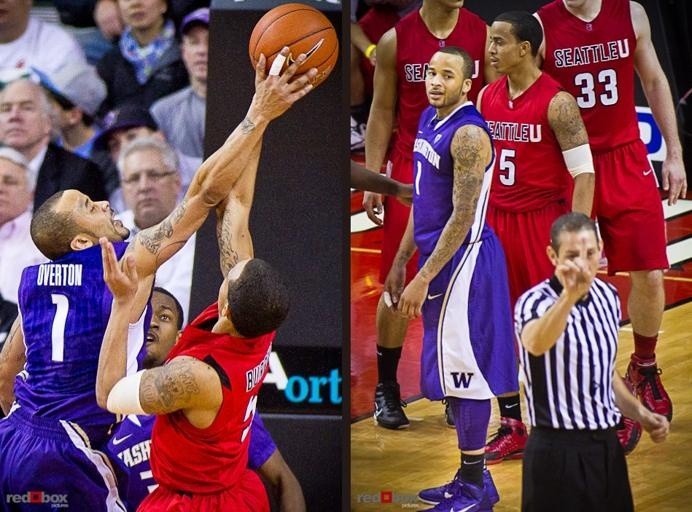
[
  {"xmin": 31, "ymin": 63, "xmax": 107, "ymax": 117},
  {"xmin": 92, "ymin": 107, "xmax": 158, "ymax": 151},
  {"xmin": 181, "ymin": 8, "xmax": 210, "ymax": 33}
]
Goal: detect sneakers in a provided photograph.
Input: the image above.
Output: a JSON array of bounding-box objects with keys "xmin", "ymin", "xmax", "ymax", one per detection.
[
  {"xmin": 445, "ymin": 401, "xmax": 456, "ymax": 428},
  {"xmin": 627, "ymin": 354, "xmax": 672, "ymax": 422},
  {"xmin": 615, "ymin": 377, "xmax": 642, "ymax": 455},
  {"xmin": 485, "ymin": 416, "xmax": 528, "ymax": 465},
  {"xmin": 415, "ymin": 470, "xmax": 499, "ymax": 512},
  {"xmin": 350, "ymin": 115, "xmax": 367, "ymax": 150},
  {"xmin": 373, "ymin": 383, "xmax": 409, "ymax": 429}
]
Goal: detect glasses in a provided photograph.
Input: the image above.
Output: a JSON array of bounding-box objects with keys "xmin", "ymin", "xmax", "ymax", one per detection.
[{"xmin": 123, "ymin": 171, "xmax": 174, "ymax": 183}]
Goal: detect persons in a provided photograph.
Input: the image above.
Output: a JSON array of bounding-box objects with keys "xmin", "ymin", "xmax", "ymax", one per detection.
[
  {"xmin": 361, "ymin": 1, "xmax": 505, "ymax": 430},
  {"xmin": 531, "ymin": 0, "xmax": 688, "ymax": 423},
  {"xmin": 1, "ymin": 0, "xmax": 211, "ymax": 349},
  {"xmin": 104, "ymin": 288, "xmax": 308, "ymax": 512},
  {"xmin": 350, "ymin": 161, "xmax": 416, "ymax": 209},
  {"xmin": 513, "ymin": 210, "xmax": 671, "ymax": 512},
  {"xmin": 351, "ymin": 22, "xmax": 380, "ymax": 116},
  {"xmin": 0, "ymin": 42, "xmax": 319, "ymax": 511},
  {"xmin": 383, "ymin": 46, "xmax": 516, "ymax": 512},
  {"xmin": 474, "ymin": 10, "xmax": 642, "ymax": 467},
  {"xmin": 93, "ymin": 133, "xmax": 290, "ymax": 511}
]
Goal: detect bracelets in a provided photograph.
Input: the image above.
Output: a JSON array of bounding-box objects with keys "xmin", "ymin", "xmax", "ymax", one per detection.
[{"xmin": 365, "ymin": 40, "xmax": 377, "ymax": 61}]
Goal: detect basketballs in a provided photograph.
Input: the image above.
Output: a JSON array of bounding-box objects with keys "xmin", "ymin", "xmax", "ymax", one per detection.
[{"xmin": 249, "ymin": 3, "xmax": 338, "ymax": 92}]
